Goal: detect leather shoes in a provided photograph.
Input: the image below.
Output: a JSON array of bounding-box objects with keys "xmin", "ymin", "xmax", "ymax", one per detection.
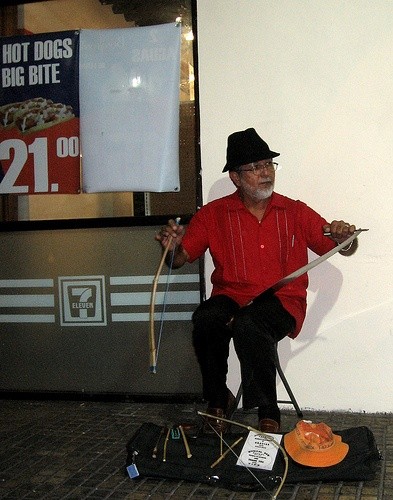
[
  {"xmin": 202, "ymin": 389, "xmax": 236, "ymax": 435},
  {"xmin": 260, "ymin": 419, "xmax": 280, "ymax": 433}
]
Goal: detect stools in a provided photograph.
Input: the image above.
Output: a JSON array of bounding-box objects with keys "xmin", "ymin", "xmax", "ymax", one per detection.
[{"xmin": 217, "ymin": 319, "xmax": 303, "ymax": 434}]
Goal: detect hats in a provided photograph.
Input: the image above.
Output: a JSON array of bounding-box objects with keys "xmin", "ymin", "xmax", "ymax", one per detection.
[
  {"xmin": 282, "ymin": 420, "xmax": 349, "ymax": 468},
  {"xmin": 222, "ymin": 128, "xmax": 281, "ymax": 172}
]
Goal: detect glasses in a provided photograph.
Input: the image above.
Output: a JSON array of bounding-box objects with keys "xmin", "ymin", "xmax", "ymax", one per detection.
[{"xmin": 238, "ymin": 162, "xmax": 278, "ymax": 173}]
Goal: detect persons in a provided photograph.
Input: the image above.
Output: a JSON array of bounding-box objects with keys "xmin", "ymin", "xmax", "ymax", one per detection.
[{"xmin": 154, "ymin": 127, "xmax": 359, "ymax": 435}]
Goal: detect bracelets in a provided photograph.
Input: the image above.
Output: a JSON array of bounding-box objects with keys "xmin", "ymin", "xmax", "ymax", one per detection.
[{"xmin": 336, "ymin": 240, "xmax": 354, "ymax": 252}]
